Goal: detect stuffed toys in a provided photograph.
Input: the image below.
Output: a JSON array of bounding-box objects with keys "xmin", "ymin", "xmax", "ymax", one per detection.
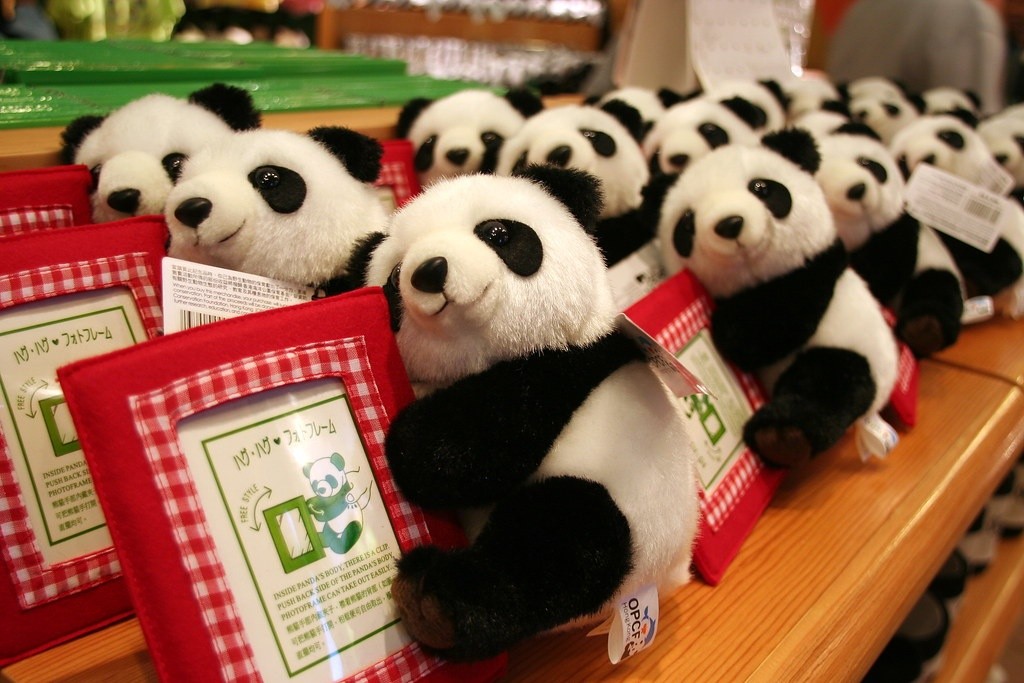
[{"xmin": 60, "ymin": 67, "xmax": 1022, "ymax": 661}]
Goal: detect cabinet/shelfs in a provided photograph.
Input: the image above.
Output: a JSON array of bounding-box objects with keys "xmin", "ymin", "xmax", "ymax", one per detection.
[{"xmin": 1, "ymin": 349, "xmax": 1024, "ymax": 683}]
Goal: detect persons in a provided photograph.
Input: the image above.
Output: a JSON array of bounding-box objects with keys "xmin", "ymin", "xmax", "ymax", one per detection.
[{"xmin": 801, "ymin": 0, "xmax": 1008, "ymax": 117}]
